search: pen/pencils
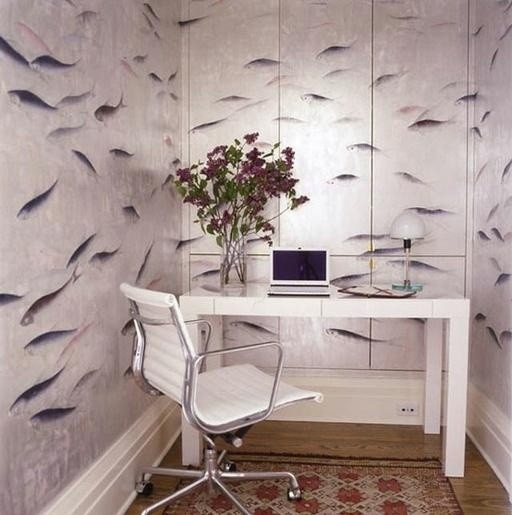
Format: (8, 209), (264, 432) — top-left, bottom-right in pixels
(373, 286), (392, 294)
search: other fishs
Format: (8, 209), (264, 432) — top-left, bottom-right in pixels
(0, 0), (512, 432)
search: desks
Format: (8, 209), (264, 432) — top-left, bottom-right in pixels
(178, 280), (472, 479)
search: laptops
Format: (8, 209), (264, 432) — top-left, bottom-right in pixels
(267, 247), (330, 296)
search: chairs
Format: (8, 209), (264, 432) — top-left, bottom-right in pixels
(117, 284), (326, 515)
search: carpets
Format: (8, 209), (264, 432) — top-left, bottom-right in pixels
(163, 448), (464, 515)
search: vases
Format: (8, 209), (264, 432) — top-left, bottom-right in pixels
(220, 239), (248, 285)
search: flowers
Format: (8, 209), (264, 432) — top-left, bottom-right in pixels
(172, 131), (310, 282)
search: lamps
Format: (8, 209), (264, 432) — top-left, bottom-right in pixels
(388, 212), (428, 291)
